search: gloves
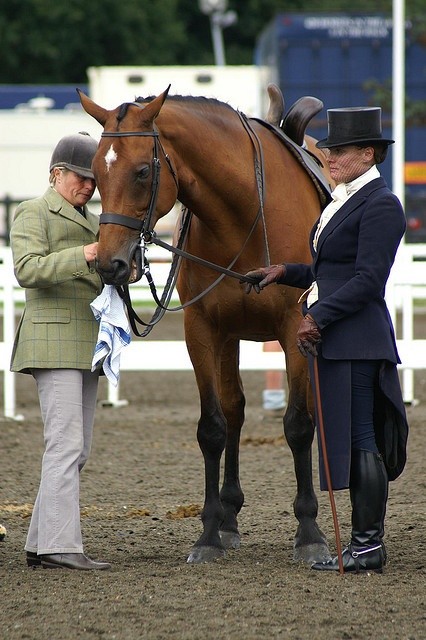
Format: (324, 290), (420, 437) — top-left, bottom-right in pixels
(295, 314), (321, 360)
(239, 264), (287, 295)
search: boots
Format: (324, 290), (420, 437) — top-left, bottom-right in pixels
(381, 462), (388, 566)
(312, 449), (386, 575)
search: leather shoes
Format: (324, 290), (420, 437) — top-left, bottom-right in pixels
(26, 551), (42, 567)
(40, 554), (112, 572)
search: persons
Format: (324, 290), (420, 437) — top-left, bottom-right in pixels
(240, 107), (410, 574)
(9, 130), (111, 572)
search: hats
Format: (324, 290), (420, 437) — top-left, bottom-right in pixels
(315, 106), (394, 150)
(48, 131), (99, 178)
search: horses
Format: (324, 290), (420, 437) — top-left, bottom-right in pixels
(75, 84), (339, 564)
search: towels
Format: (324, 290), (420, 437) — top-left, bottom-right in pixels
(90, 285), (133, 387)
